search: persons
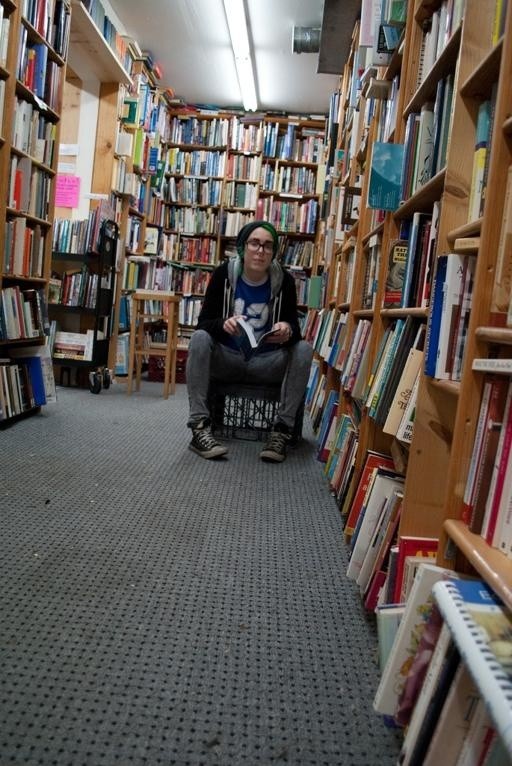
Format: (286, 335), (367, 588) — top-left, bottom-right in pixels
(185, 221), (313, 463)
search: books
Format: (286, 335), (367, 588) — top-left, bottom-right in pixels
(1, 0), (177, 419)
(178, 99), (307, 357)
(306, 0), (512, 766)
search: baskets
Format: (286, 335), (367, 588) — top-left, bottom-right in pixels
(208, 382), (308, 448)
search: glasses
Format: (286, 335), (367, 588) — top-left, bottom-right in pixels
(246, 240), (274, 253)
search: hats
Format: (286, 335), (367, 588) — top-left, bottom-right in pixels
(236, 221), (279, 260)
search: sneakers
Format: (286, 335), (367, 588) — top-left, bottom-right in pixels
(260, 423), (293, 462)
(189, 419), (229, 459)
